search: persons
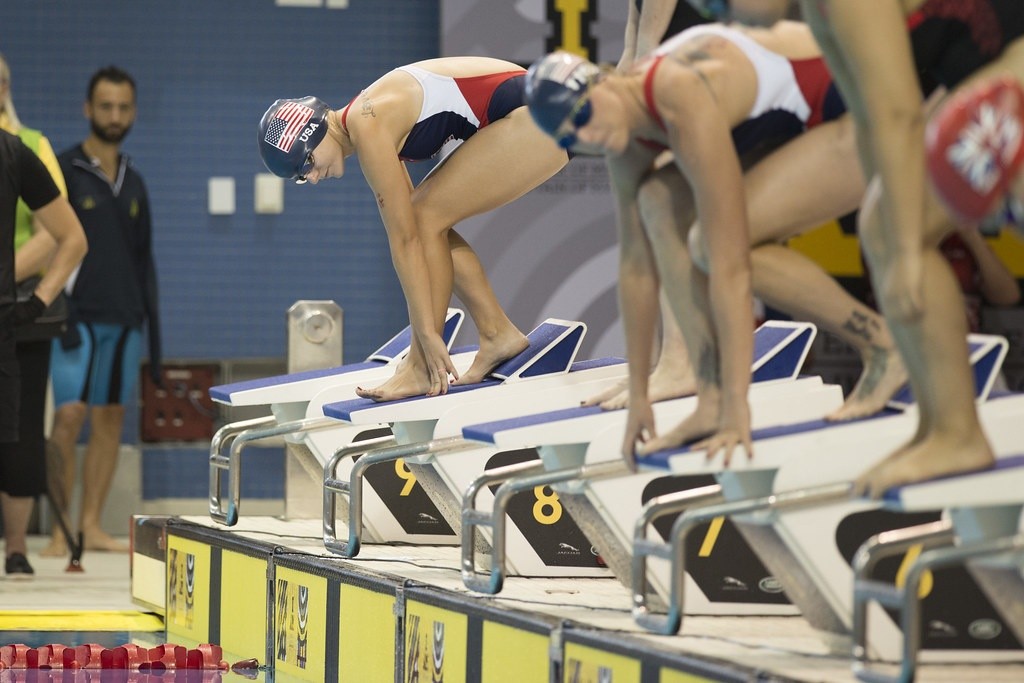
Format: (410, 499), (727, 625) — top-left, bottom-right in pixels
(578, 0), (803, 409)
(257, 55), (572, 403)
(41, 64), (160, 557)
(687, 0), (1024, 500)
(0, 56), (88, 579)
(525, 19), (909, 466)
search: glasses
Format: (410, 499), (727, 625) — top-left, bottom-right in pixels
(296, 154), (315, 186)
(559, 99), (592, 148)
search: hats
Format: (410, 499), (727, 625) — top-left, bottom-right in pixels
(256, 96), (331, 179)
(522, 48), (599, 139)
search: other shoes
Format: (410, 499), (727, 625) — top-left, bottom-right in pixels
(4, 552), (33, 582)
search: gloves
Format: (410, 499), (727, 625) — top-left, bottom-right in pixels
(0, 292), (47, 328)
